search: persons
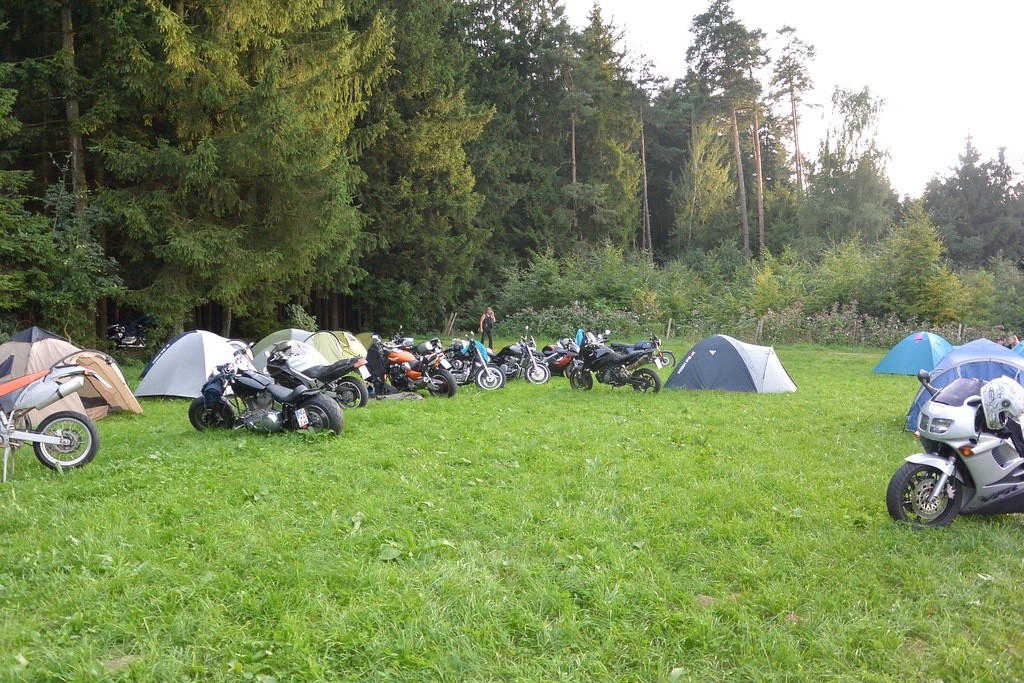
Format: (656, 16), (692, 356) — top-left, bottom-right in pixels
(480, 307), (497, 349)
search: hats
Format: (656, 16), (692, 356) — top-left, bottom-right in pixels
(995, 336), (1003, 340)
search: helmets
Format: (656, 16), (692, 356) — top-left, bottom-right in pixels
(980, 375), (1024, 430)
(455, 342), (462, 351)
(431, 338), (443, 348)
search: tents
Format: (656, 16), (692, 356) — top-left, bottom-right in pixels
(0, 326), (144, 423)
(903, 338), (1024, 433)
(871, 331), (955, 377)
(251, 328), (382, 373)
(132, 329), (258, 399)
(663, 334), (799, 395)
(1011, 339), (1024, 359)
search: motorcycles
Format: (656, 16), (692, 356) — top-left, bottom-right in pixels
(414, 331), (506, 390)
(264, 341), (368, 410)
(0, 364), (112, 483)
(486, 326), (551, 385)
(886, 369), (1024, 530)
(544, 327), (611, 379)
(389, 325), (442, 359)
(372, 335), (457, 398)
(569, 329), (676, 396)
(188, 342), (344, 438)
(611, 332), (676, 369)
